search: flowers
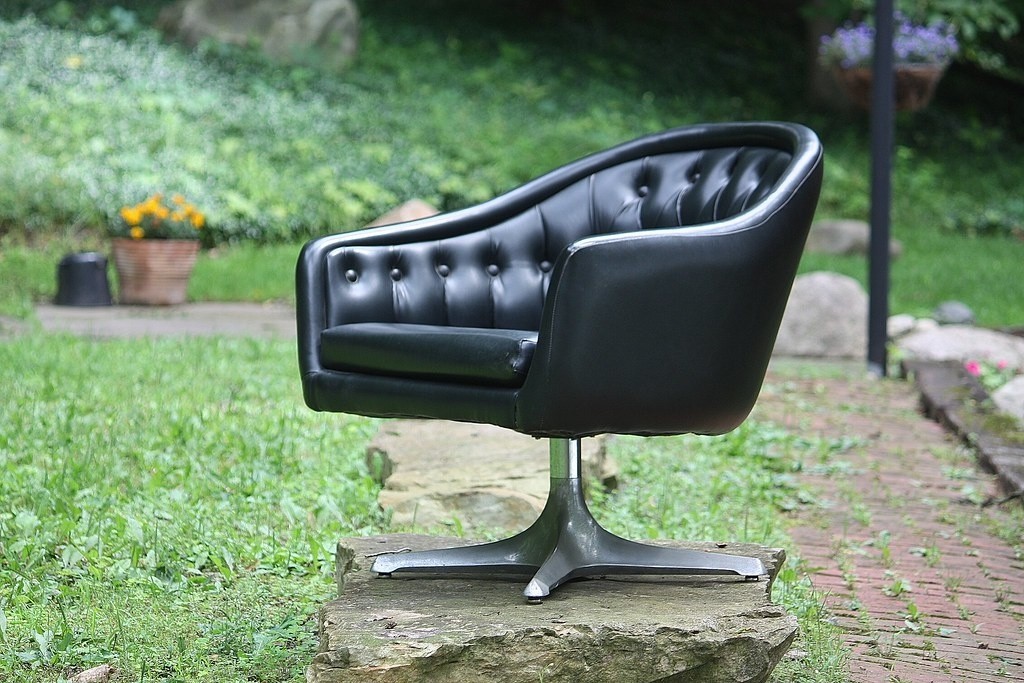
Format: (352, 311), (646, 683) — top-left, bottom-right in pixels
(820, 22), (958, 68)
(102, 184), (209, 240)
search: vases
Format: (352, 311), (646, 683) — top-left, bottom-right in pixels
(111, 237), (202, 303)
(831, 67), (936, 111)
(54, 255), (113, 305)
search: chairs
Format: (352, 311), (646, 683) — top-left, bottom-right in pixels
(295, 121), (823, 603)
